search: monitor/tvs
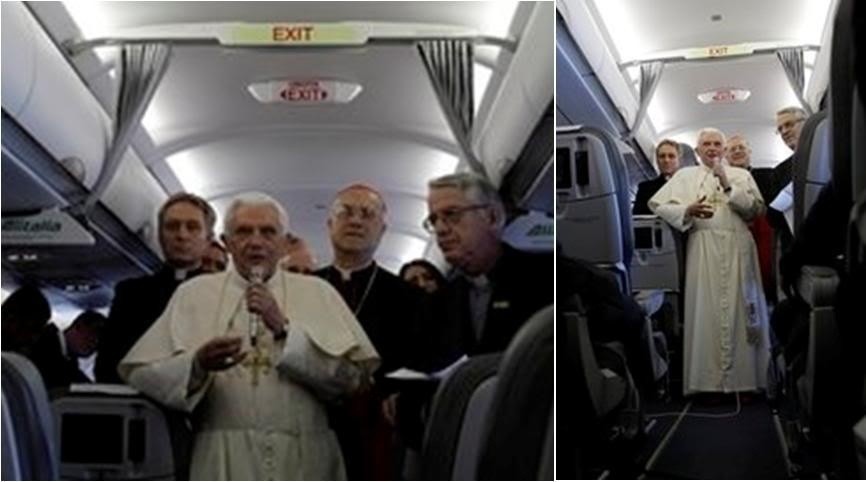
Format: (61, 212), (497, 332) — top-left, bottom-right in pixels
(57, 410), (127, 469)
(555, 143), (573, 190)
(633, 225), (653, 250)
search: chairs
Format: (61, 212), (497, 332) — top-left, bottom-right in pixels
(2, 350), (61, 481)
(421, 306), (555, 480)
(47, 380), (191, 481)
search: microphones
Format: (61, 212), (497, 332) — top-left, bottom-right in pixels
(245, 268), (265, 343)
(712, 157), (721, 190)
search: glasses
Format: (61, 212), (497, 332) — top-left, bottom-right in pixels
(423, 204), (489, 234)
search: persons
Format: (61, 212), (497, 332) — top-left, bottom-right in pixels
(633, 138), (683, 251)
(770, 107), (808, 180)
(1, 173), (552, 481)
(727, 135), (790, 256)
(648, 128), (772, 414)
